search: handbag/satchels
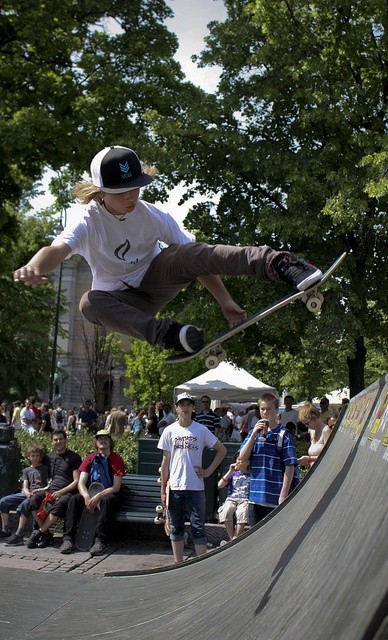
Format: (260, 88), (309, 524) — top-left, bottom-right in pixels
(36, 491), (56, 522)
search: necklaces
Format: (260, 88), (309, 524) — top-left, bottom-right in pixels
(104, 203), (126, 221)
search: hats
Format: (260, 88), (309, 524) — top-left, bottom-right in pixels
(90, 146), (152, 195)
(175, 391), (195, 405)
(96, 430), (111, 437)
(233, 449), (252, 458)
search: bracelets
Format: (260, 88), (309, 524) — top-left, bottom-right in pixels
(307, 456), (311, 464)
(221, 477), (228, 482)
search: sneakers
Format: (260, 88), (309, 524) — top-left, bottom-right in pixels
(163, 322), (204, 353)
(36, 533), (54, 548)
(26, 530), (41, 547)
(0, 530), (11, 538)
(2, 533), (25, 545)
(277, 254), (323, 291)
(59, 534), (75, 554)
(89, 538), (110, 556)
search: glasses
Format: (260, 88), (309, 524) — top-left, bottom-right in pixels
(202, 400), (209, 404)
(305, 420), (311, 425)
(85, 403), (90, 406)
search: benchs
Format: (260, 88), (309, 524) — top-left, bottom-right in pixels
(114, 474), (160, 525)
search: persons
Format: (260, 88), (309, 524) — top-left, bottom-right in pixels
(318, 397), (333, 424)
(14, 146), (323, 354)
(0, 445), (50, 546)
(285, 422), (298, 440)
(297, 422), (310, 443)
(327, 414), (337, 431)
(194, 395), (221, 437)
(59, 429), (126, 556)
(278, 396), (299, 435)
(24, 429), (82, 548)
(214, 403), (259, 439)
(218, 449), (252, 542)
(237, 393), (299, 528)
(157, 392), (227, 564)
(297, 401), (331, 468)
(0, 395), (179, 437)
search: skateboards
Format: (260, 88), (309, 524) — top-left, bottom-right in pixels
(153, 466), (171, 537)
(75, 481), (105, 552)
(159, 251), (348, 366)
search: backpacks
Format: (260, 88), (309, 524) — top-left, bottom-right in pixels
(90, 453), (112, 489)
(56, 410), (62, 423)
(277, 428), (299, 494)
(224, 416), (233, 437)
